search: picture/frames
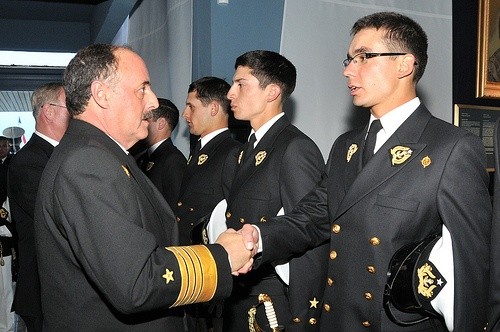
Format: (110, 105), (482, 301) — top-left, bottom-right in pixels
(454, 104), (500, 173)
(476, 0), (500, 100)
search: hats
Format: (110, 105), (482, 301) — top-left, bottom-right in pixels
(382, 224), (457, 332)
(271, 206), (294, 285)
(3, 127), (25, 138)
(189, 199), (229, 246)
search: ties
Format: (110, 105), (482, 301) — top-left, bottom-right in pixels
(243, 133), (257, 163)
(193, 141), (201, 158)
(362, 120), (383, 169)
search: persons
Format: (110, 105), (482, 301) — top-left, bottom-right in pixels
(174, 76), (245, 332)
(2, 127), (25, 154)
(232, 12), (493, 332)
(213, 50), (326, 332)
(0, 136), (11, 205)
(483, 117), (500, 332)
(34, 43), (258, 332)
(128, 97), (188, 215)
(7, 83), (81, 332)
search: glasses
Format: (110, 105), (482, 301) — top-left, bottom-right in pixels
(342, 53), (418, 70)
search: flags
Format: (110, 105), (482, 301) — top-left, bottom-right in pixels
(17, 117), (26, 143)
